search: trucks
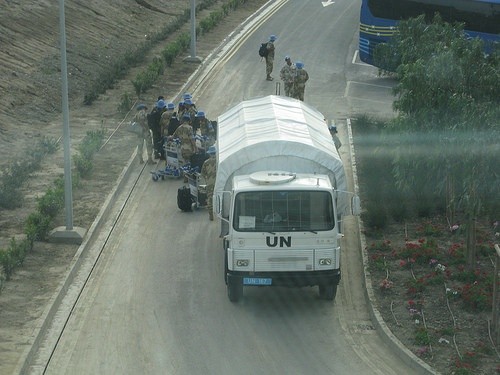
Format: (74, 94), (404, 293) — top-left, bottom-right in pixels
(212, 95), (362, 302)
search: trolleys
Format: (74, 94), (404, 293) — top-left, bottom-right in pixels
(185, 169), (207, 208)
(151, 142), (181, 181)
(183, 137), (206, 184)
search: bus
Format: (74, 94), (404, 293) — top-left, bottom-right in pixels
(359, 0), (500, 76)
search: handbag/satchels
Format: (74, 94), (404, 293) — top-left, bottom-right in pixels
(127, 121), (143, 136)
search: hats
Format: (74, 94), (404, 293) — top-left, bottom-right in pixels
(329, 126), (337, 132)
(206, 146), (216, 154)
(179, 101), (186, 106)
(182, 93), (193, 98)
(137, 103), (148, 111)
(185, 99), (192, 105)
(269, 35), (277, 41)
(157, 100), (166, 108)
(285, 56), (290, 62)
(167, 103), (176, 109)
(296, 62), (304, 67)
(195, 111), (206, 117)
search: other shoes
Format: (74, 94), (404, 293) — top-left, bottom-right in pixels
(210, 218), (213, 221)
(266, 76), (274, 81)
(140, 161), (144, 164)
(155, 154), (160, 159)
(184, 177), (188, 183)
(148, 160), (157, 165)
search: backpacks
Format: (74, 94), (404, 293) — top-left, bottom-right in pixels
(259, 43), (268, 57)
(161, 111), (171, 129)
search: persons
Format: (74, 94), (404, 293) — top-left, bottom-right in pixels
(169, 116), (199, 183)
(148, 93), (202, 160)
(279, 55), (298, 98)
(264, 35), (278, 81)
(291, 62), (310, 102)
(201, 146), (218, 222)
(130, 103), (157, 165)
(194, 111), (215, 158)
(328, 124), (342, 150)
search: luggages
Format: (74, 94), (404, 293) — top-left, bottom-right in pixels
(177, 185), (191, 210)
